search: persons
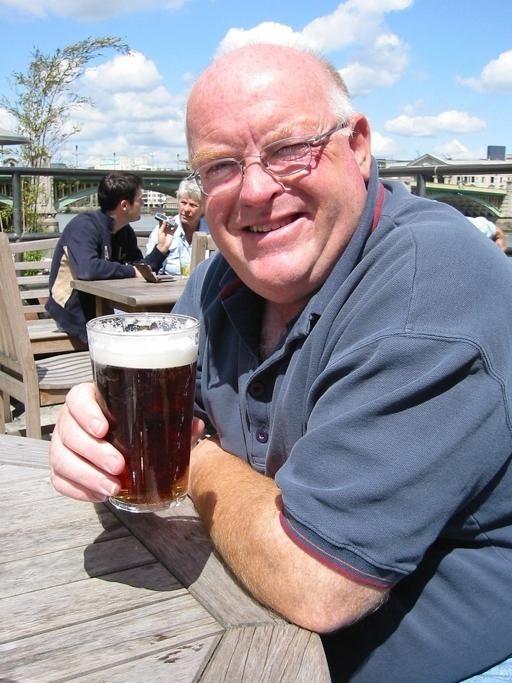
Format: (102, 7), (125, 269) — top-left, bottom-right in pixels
(463, 214), (508, 252)
(46, 39), (511, 683)
(40, 168), (178, 353)
(144, 174), (207, 277)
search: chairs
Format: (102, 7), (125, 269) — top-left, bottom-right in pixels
(190, 231), (218, 274)
(0, 233), (94, 440)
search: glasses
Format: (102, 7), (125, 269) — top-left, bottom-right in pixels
(186, 119), (352, 197)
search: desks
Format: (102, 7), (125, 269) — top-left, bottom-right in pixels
(0, 434), (332, 683)
(69, 274), (191, 318)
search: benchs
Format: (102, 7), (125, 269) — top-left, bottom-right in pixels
(9, 237), (150, 360)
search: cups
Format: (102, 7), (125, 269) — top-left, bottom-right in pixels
(86, 313), (201, 513)
(179, 246), (192, 275)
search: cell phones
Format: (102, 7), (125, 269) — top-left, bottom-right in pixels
(154, 212), (176, 227)
